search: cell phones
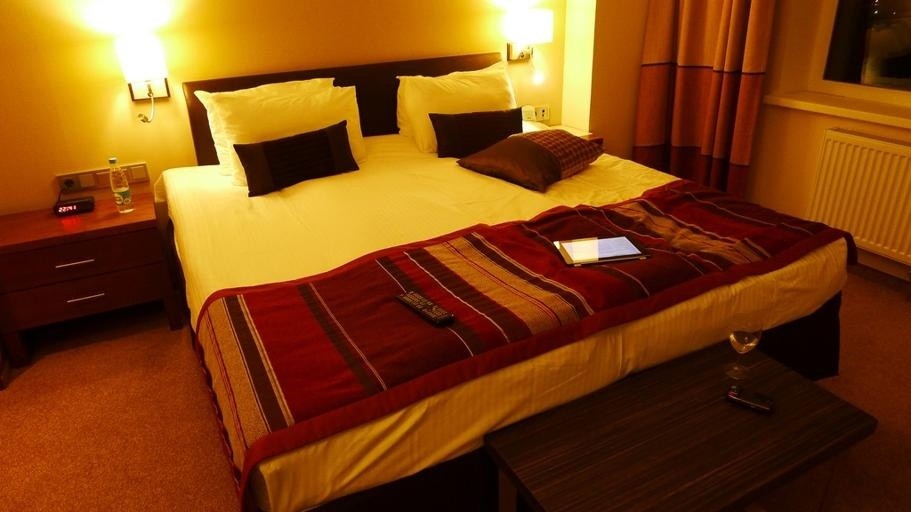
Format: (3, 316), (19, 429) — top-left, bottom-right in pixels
(726, 385), (776, 412)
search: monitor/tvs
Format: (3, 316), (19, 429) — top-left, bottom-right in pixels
(823, 0), (911, 93)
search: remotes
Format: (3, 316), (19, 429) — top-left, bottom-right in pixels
(396, 291), (455, 327)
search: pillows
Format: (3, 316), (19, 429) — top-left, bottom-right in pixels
(211, 86), (366, 185)
(429, 108), (523, 159)
(394, 59), (516, 155)
(458, 127), (607, 192)
(194, 78), (335, 180)
(232, 119), (360, 198)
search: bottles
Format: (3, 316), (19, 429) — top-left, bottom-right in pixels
(105, 157), (135, 213)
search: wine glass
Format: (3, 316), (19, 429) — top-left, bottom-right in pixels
(723, 310), (765, 382)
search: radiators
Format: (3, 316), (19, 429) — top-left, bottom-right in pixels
(809, 128), (911, 267)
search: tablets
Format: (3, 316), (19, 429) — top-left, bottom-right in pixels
(553, 235), (650, 267)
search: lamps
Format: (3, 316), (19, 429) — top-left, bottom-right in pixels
(116, 40), (171, 127)
(502, 11), (557, 63)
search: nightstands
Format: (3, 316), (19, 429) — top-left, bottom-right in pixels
(0, 194), (183, 367)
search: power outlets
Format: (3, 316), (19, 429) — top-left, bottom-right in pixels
(58, 173), (80, 196)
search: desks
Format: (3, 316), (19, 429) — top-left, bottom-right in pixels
(486, 345), (880, 512)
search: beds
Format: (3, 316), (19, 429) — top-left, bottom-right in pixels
(154, 51), (860, 512)
